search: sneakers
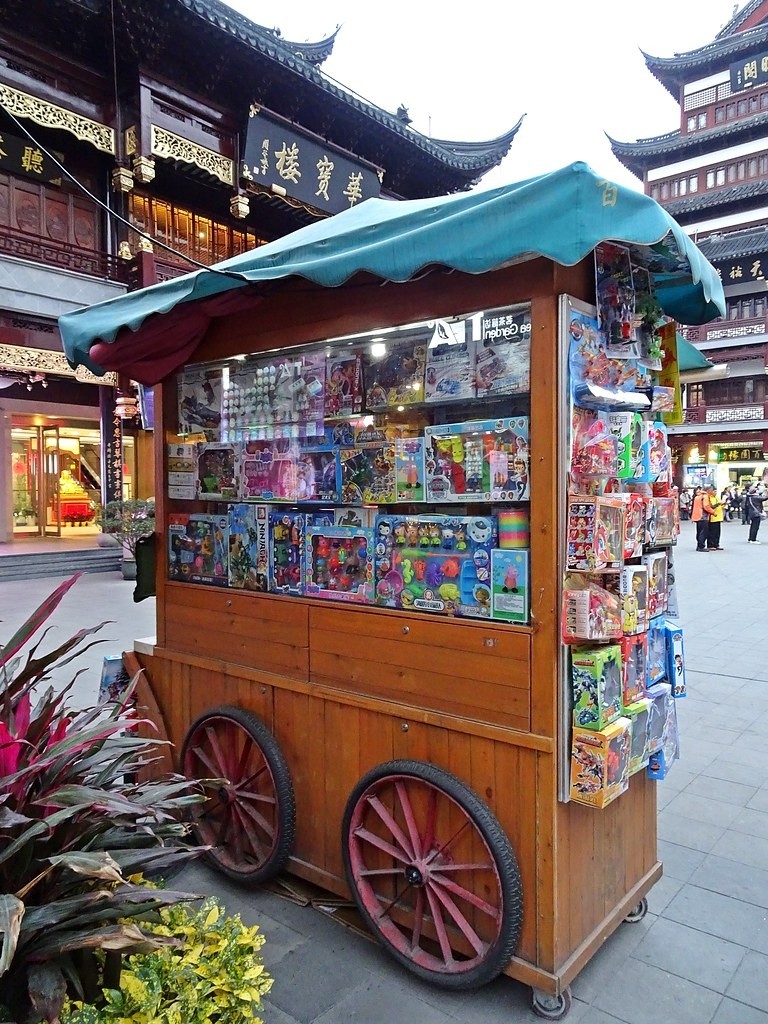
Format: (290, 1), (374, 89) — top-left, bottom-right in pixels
(696, 547), (710, 552)
(750, 540), (761, 545)
(707, 547), (716, 551)
(747, 539), (759, 543)
(716, 547), (724, 550)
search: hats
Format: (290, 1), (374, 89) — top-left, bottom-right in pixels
(745, 484), (752, 490)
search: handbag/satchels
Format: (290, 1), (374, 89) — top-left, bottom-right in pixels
(759, 510), (768, 520)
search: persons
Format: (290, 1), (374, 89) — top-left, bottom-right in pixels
(691, 486), (717, 552)
(721, 483), (768, 545)
(705, 484), (729, 551)
(679, 486), (702, 520)
